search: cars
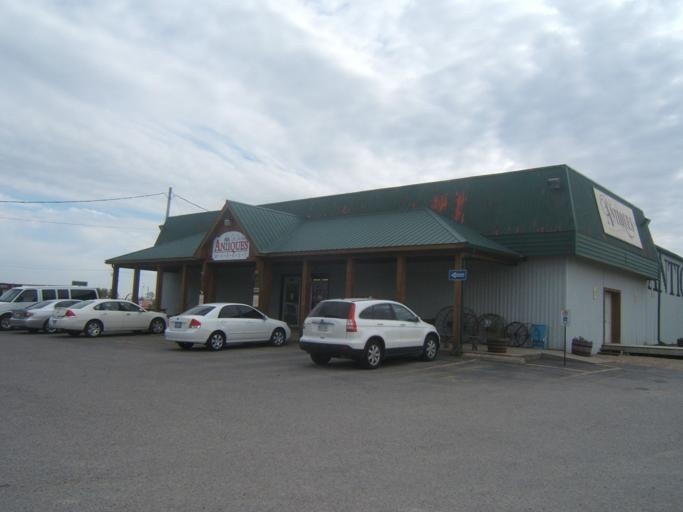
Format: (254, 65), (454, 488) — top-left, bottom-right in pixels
(49, 298), (168, 341)
(10, 299), (83, 332)
(162, 299), (290, 355)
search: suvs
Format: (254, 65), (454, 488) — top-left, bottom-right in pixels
(296, 295), (446, 366)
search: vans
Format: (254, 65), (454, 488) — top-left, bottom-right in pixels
(0, 285), (98, 331)
